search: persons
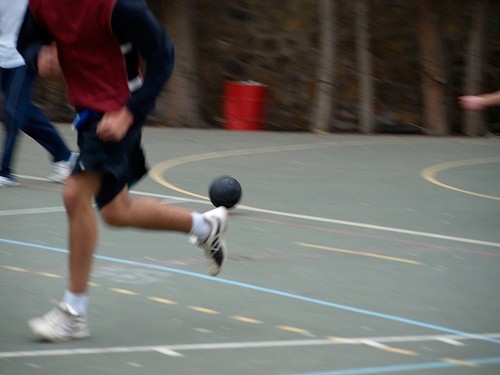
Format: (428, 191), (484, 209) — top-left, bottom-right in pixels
(458, 91), (500, 112)
(0, 0), (78, 188)
(16, 0), (228, 342)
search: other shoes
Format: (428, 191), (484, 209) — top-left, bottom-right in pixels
(0, 175), (21, 188)
(49, 152), (80, 183)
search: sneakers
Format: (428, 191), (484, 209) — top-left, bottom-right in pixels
(25, 297), (91, 340)
(191, 205), (228, 275)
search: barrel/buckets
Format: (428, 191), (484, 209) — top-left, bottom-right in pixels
(223, 79), (266, 128)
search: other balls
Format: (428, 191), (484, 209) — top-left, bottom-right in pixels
(209, 175), (241, 209)
